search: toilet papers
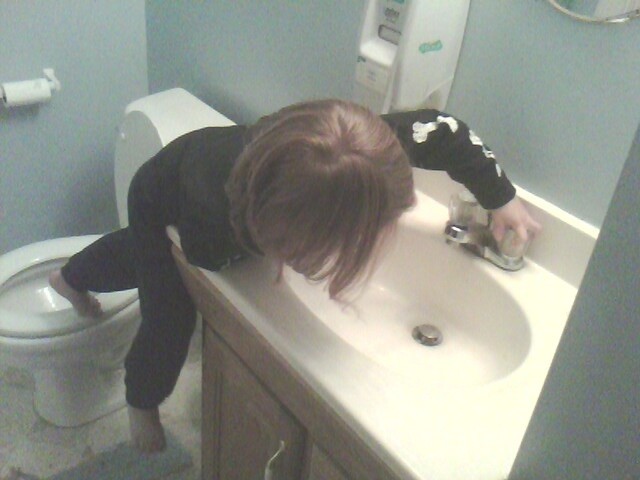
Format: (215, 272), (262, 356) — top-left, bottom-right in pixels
(0, 68), (61, 107)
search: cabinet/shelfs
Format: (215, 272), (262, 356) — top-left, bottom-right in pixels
(202, 322), (400, 480)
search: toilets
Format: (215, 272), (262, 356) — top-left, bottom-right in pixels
(0, 86), (237, 428)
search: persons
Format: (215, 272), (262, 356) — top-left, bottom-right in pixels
(49, 97), (543, 459)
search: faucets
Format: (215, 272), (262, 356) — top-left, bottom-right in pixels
(444, 198), (529, 270)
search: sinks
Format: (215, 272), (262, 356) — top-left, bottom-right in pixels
(278, 216), (534, 385)
